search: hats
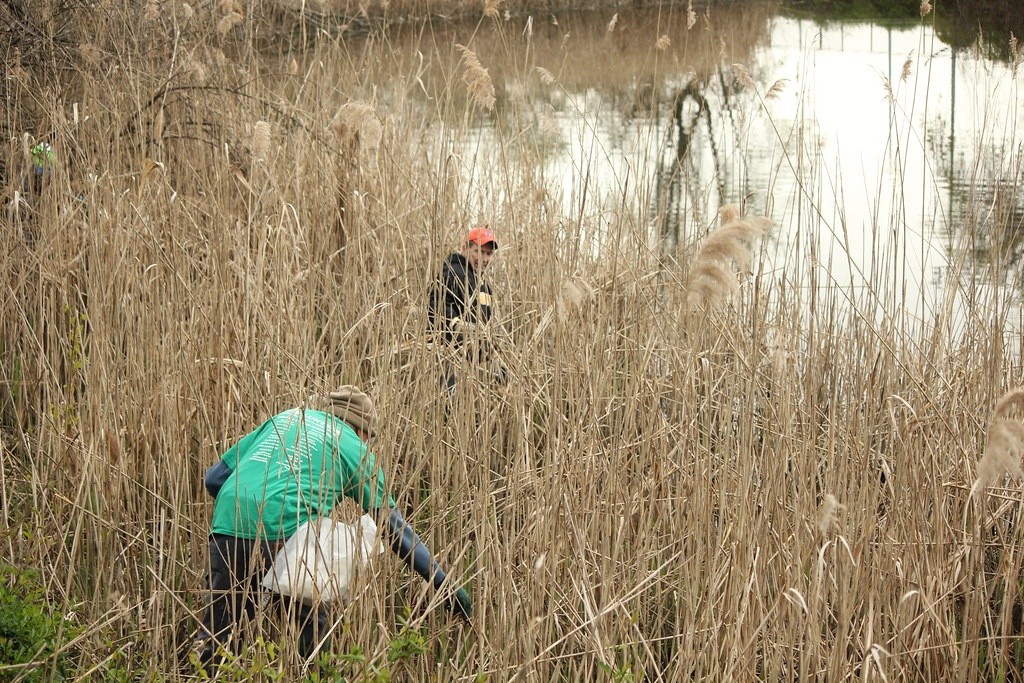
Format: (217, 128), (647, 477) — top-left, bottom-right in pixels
(329, 384), (378, 436)
(465, 228), (498, 250)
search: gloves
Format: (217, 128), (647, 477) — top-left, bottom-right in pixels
(438, 578), (474, 620)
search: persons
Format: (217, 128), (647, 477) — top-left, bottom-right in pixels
(194, 386), (471, 683)
(27, 142), (63, 203)
(392, 229), (508, 519)
